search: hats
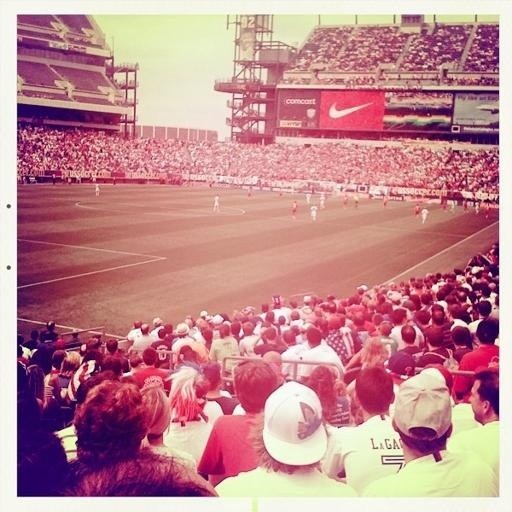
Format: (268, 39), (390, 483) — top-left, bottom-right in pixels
(392, 367), (452, 441)
(262, 381), (328, 466)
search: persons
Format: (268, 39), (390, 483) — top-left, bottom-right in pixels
(427, 242), (499, 292)
(17, 122), (295, 187)
(290, 141), (499, 224)
(260, 277), (499, 365)
(17, 309), (499, 495)
(213, 194), (221, 212)
(284, 23), (498, 86)
(95, 183), (101, 196)
